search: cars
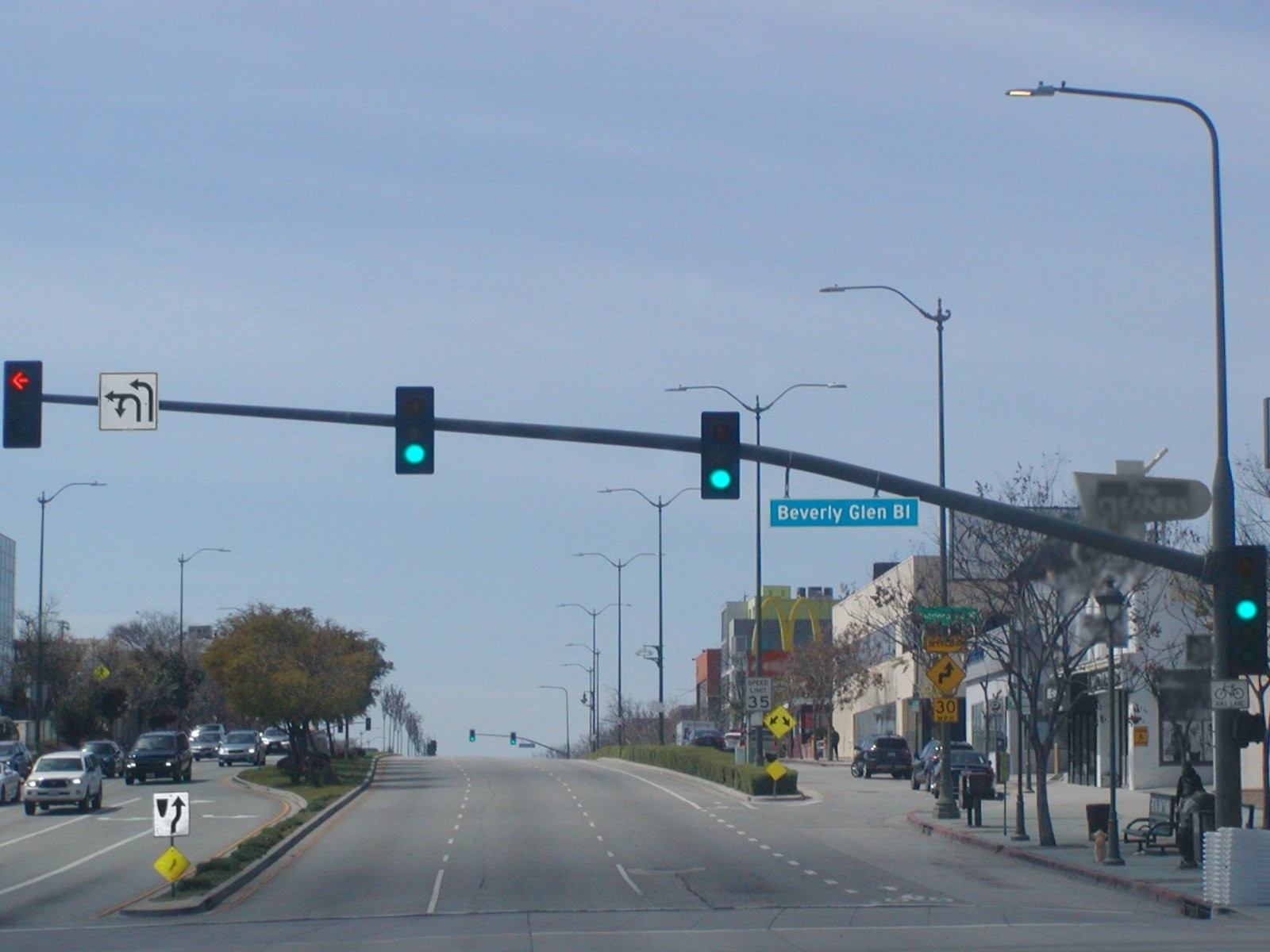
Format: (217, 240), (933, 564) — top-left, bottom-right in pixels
(686, 729), (727, 752)
(0, 723), (292, 817)
(848, 732), (997, 805)
(723, 733), (740, 750)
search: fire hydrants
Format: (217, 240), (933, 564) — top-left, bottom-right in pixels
(1091, 829), (1110, 862)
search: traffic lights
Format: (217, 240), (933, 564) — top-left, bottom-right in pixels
(510, 732), (516, 745)
(700, 411), (743, 502)
(470, 729), (475, 742)
(1, 360), (44, 449)
(1212, 545), (1270, 679)
(395, 386), (436, 475)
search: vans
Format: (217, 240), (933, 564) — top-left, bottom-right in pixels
(747, 728), (777, 758)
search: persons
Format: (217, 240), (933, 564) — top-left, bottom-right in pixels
(1174, 762), (1206, 820)
(1171, 723), (1203, 762)
(829, 727), (840, 760)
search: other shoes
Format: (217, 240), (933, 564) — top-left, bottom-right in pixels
(1178, 860), (1198, 869)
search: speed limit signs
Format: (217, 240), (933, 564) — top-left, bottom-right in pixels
(745, 678), (771, 711)
(933, 697), (961, 724)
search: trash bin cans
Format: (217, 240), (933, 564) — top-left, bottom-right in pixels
(958, 770), (974, 808)
(1086, 804), (1110, 841)
(1192, 811), (1215, 863)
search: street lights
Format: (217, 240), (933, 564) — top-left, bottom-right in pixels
(573, 552), (667, 748)
(177, 547), (232, 734)
(666, 383), (849, 772)
(820, 283), (962, 822)
(1089, 572), (1132, 865)
(538, 603), (633, 752)
(597, 488), (701, 747)
(1005, 80), (1241, 838)
(1009, 610), (1033, 840)
(35, 481), (108, 750)
